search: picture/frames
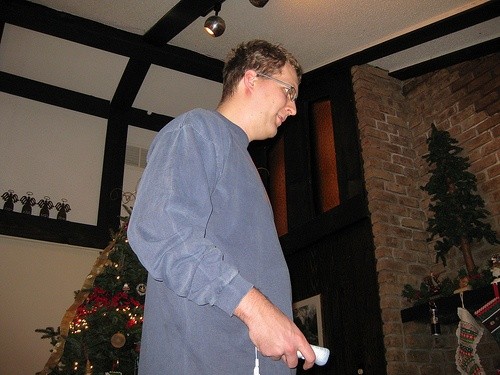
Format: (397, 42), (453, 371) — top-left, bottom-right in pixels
(293, 294), (324, 348)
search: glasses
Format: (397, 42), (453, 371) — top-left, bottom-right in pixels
(256, 72), (297, 102)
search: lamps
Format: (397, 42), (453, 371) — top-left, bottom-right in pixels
(204, 2), (226, 38)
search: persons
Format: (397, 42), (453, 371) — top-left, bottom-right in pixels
(127, 40), (315, 375)
